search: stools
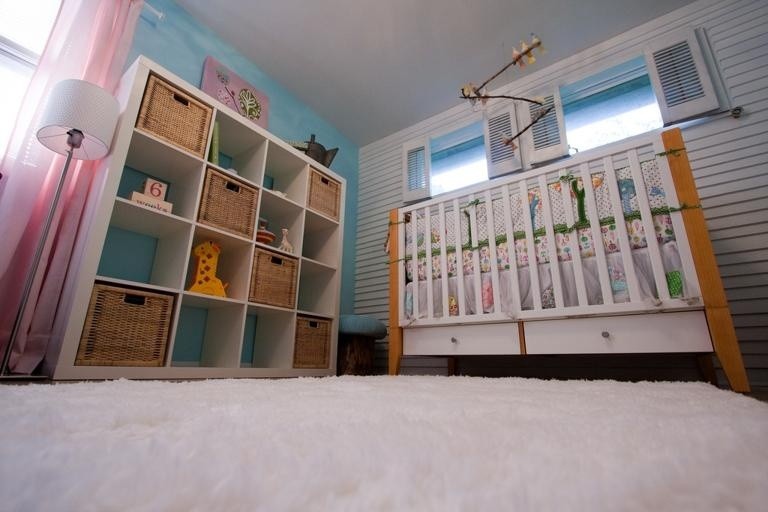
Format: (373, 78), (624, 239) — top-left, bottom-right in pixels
(338, 313), (387, 374)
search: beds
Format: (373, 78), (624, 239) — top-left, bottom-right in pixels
(384, 127), (751, 392)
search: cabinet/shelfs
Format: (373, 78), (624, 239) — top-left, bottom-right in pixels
(44, 55), (349, 378)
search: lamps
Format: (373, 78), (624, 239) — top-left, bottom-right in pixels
(3, 77), (124, 379)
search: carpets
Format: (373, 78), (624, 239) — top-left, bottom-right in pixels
(2, 371), (768, 512)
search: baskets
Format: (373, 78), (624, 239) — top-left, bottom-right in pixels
(306, 165), (340, 223)
(197, 168), (259, 241)
(293, 316), (332, 369)
(249, 247), (299, 310)
(135, 75), (214, 159)
(75, 283), (174, 368)
